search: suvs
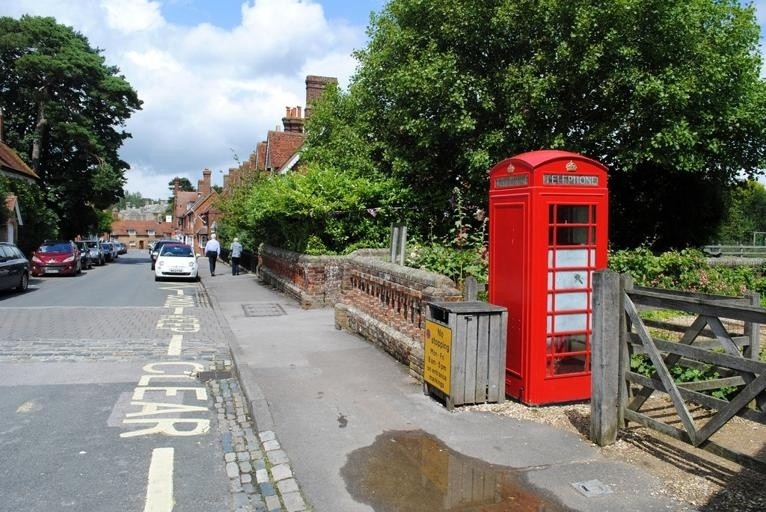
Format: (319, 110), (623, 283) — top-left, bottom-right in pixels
(0, 241), (31, 295)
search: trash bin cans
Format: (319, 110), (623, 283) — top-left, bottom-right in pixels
(423, 301), (508, 410)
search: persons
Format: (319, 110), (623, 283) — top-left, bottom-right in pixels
(204, 233), (220, 276)
(229, 238), (242, 275)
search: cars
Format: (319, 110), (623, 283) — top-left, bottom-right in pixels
(148, 238), (199, 282)
(30, 237), (128, 278)
